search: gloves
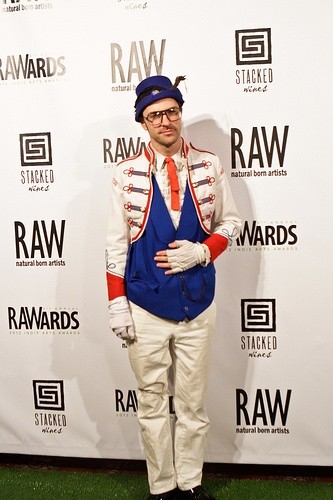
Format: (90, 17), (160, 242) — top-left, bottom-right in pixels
(109, 301), (134, 340)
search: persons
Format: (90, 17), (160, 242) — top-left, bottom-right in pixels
(105, 76), (241, 500)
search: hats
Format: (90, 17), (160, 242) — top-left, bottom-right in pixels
(134, 76), (184, 122)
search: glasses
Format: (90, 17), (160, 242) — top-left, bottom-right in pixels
(143, 107), (182, 124)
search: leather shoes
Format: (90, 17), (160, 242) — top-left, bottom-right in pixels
(177, 486), (216, 500)
(148, 487), (180, 500)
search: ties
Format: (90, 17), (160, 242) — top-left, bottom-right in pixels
(164, 158), (180, 211)
